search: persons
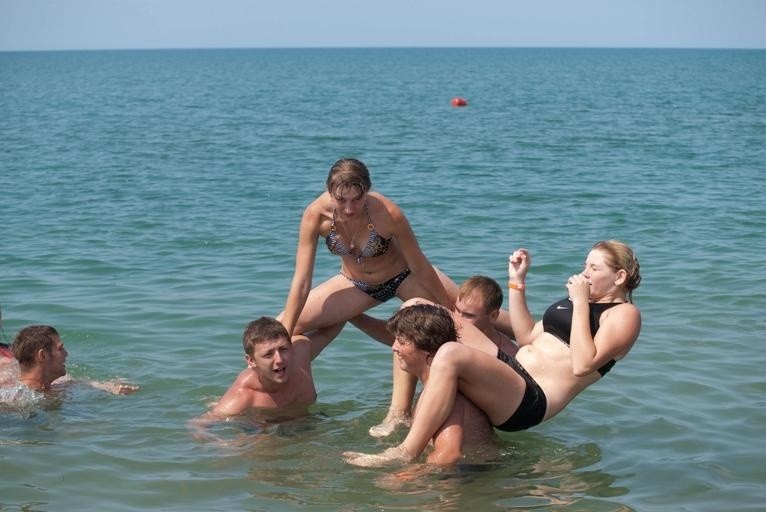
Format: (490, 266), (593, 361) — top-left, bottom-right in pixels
(188, 316), (348, 458)
(0, 322), (141, 419)
(342, 235), (643, 470)
(375, 299), (507, 490)
(346, 277), (519, 363)
(244, 156), (518, 365)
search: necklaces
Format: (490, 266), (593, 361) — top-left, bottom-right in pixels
(339, 216), (364, 251)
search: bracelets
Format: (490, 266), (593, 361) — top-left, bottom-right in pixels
(504, 281), (526, 292)
